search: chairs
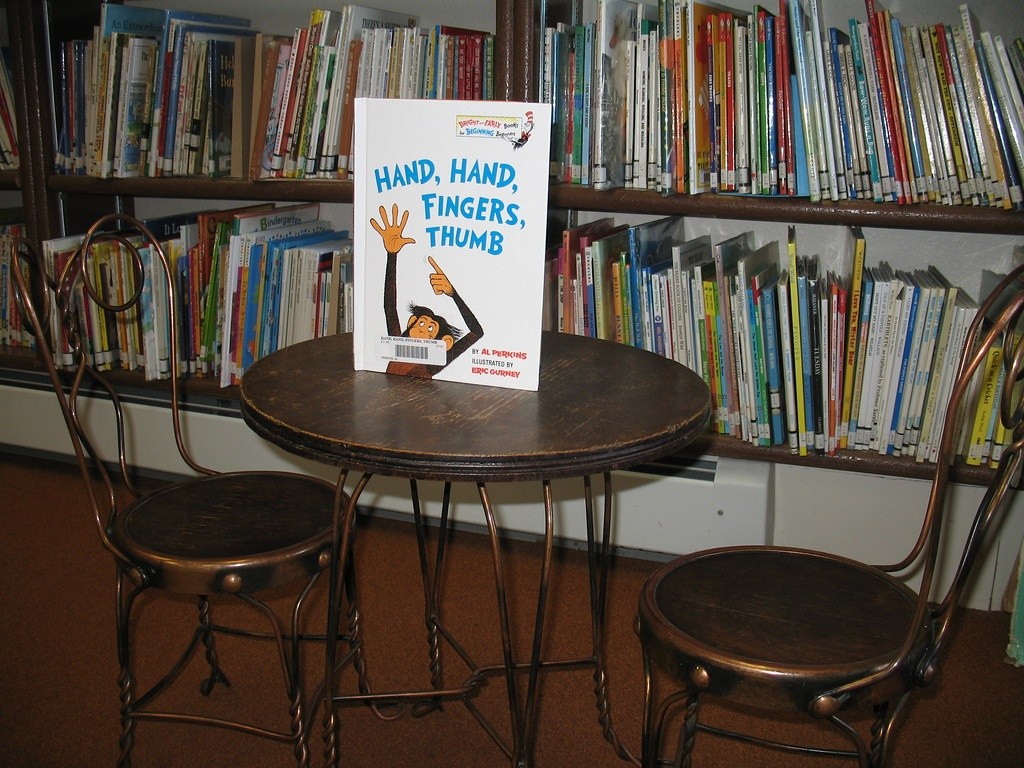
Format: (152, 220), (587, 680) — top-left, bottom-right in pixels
(632, 264), (1024, 768)
(11, 212), (406, 768)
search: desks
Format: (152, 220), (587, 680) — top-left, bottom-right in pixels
(238, 330), (712, 768)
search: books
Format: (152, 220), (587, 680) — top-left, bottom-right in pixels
(353, 98), (551, 392)
(0, 201), (355, 388)
(543, 216), (1024, 470)
(0, 0), (496, 182)
(538, 0), (1024, 211)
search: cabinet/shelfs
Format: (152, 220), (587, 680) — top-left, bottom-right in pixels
(0, 0), (1024, 492)
